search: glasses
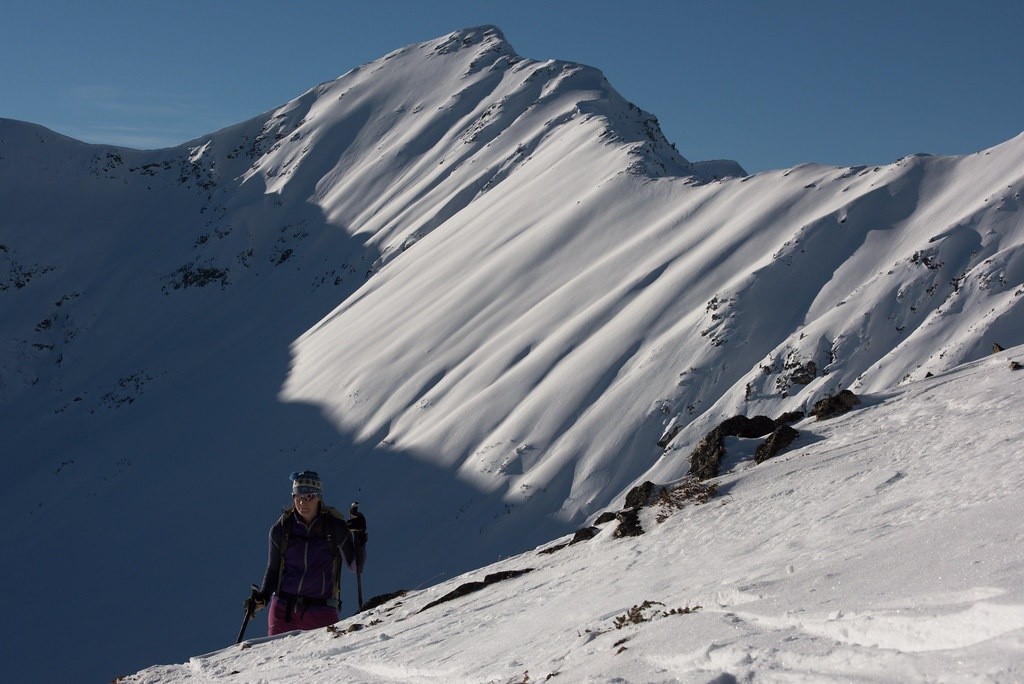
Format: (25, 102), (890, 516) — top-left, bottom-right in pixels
(293, 493), (317, 501)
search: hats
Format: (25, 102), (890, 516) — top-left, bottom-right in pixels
(289, 470), (323, 497)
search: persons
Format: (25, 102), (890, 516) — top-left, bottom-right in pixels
(243, 470), (369, 640)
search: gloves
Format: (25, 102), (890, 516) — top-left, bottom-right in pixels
(243, 595), (266, 619)
(345, 508), (369, 545)
